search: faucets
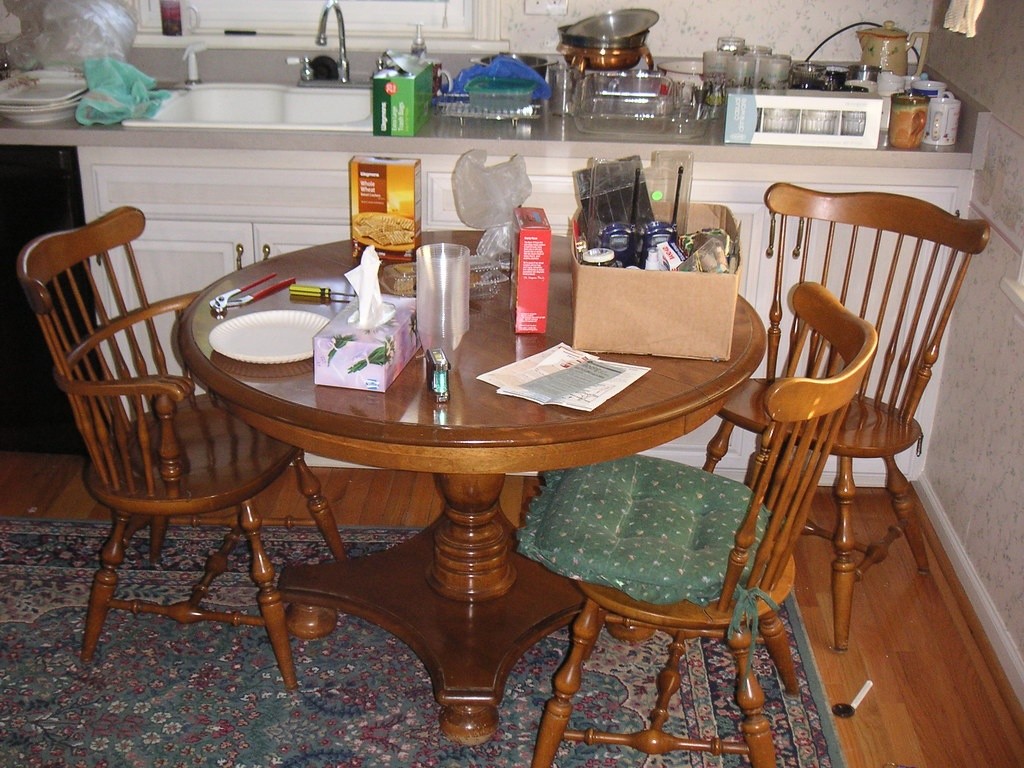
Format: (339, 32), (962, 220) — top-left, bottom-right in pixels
(286, 0), (387, 90)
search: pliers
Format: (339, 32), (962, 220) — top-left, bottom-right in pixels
(208, 272), (295, 313)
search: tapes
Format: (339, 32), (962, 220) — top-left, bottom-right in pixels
(583, 247), (615, 263)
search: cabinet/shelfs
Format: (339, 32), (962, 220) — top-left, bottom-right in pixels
(76, 145), (976, 488)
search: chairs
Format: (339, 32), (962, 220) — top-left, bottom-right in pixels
(701, 182), (990, 651)
(17, 206), (346, 694)
(517, 279), (878, 768)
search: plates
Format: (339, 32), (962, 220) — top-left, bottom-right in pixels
(351, 212), (414, 251)
(0, 70), (88, 124)
(209, 310), (331, 365)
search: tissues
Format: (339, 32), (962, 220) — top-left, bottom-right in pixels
(312, 241), (426, 394)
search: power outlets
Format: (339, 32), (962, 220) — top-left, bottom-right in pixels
(524, 0), (567, 16)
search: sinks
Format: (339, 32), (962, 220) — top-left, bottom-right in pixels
(121, 82), (374, 132)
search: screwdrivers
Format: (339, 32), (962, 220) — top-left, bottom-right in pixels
(290, 285), (356, 300)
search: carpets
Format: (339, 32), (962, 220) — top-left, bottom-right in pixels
(0, 512), (845, 767)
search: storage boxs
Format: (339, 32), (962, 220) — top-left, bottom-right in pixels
(371, 47), (435, 136)
(508, 206), (553, 335)
(568, 200), (742, 360)
(345, 156), (422, 266)
(721, 87), (883, 152)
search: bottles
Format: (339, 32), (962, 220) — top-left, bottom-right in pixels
(0, 43), (21, 81)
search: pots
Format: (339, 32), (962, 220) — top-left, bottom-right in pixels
(556, 42), (646, 71)
(557, 25), (650, 48)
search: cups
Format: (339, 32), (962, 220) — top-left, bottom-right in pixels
(160, 0), (199, 36)
(793, 62), (880, 91)
(416, 242), (470, 372)
(877, 72), (961, 149)
(703, 38), (791, 119)
(423, 58), (453, 98)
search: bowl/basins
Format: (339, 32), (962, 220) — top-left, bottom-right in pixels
(470, 54), (558, 81)
(464, 77), (537, 110)
(657, 61), (703, 86)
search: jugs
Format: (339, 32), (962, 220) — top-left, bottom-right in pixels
(856, 21), (933, 77)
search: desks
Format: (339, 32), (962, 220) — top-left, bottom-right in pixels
(171, 231), (765, 747)
